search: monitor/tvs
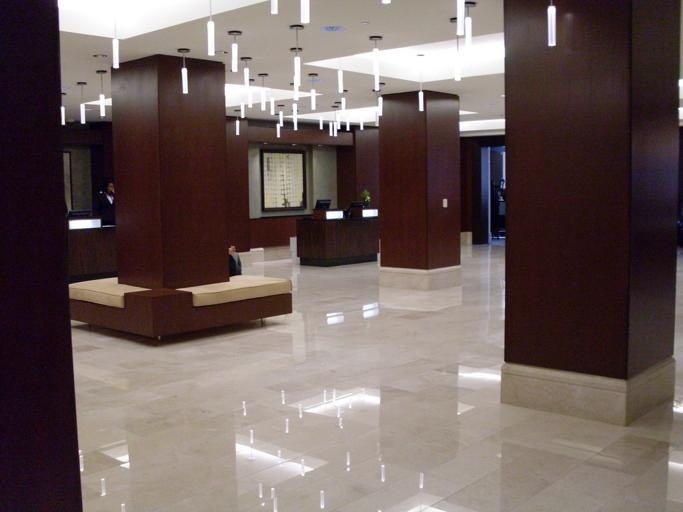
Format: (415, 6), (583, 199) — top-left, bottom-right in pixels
(315, 200), (331, 210)
(69, 210), (91, 219)
(352, 202), (362, 208)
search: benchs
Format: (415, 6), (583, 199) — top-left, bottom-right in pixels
(67, 276), (293, 344)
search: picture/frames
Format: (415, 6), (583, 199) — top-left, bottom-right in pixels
(259, 148), (306, 211)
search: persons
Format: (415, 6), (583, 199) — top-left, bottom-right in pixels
(92, 180), (115, 226)
